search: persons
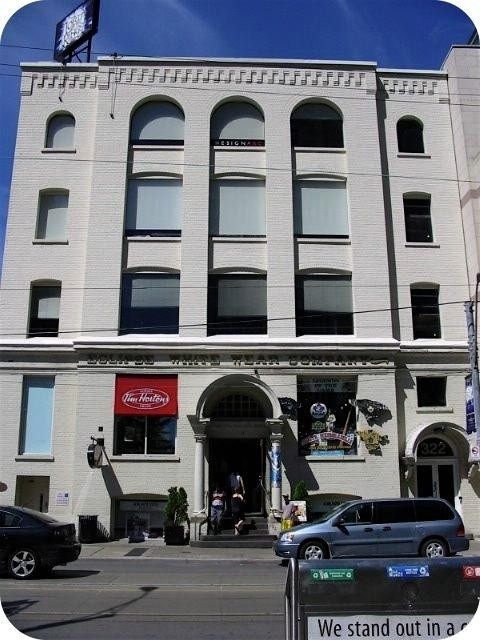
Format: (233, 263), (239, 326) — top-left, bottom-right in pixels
(211, 470), (246, 537)
(269, 493), (303, 531)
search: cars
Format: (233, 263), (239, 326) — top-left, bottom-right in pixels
(0, 506), (81, 578)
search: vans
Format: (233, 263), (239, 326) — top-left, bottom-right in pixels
(272, 498), (469, 560)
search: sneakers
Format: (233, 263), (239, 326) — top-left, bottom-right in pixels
(234, 525), (241, 536)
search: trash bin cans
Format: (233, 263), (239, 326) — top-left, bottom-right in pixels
(283, 558), (480, 640)
(78, 515), (99, 544)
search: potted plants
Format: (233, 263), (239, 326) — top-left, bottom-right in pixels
(163, 485), (190, 545)
